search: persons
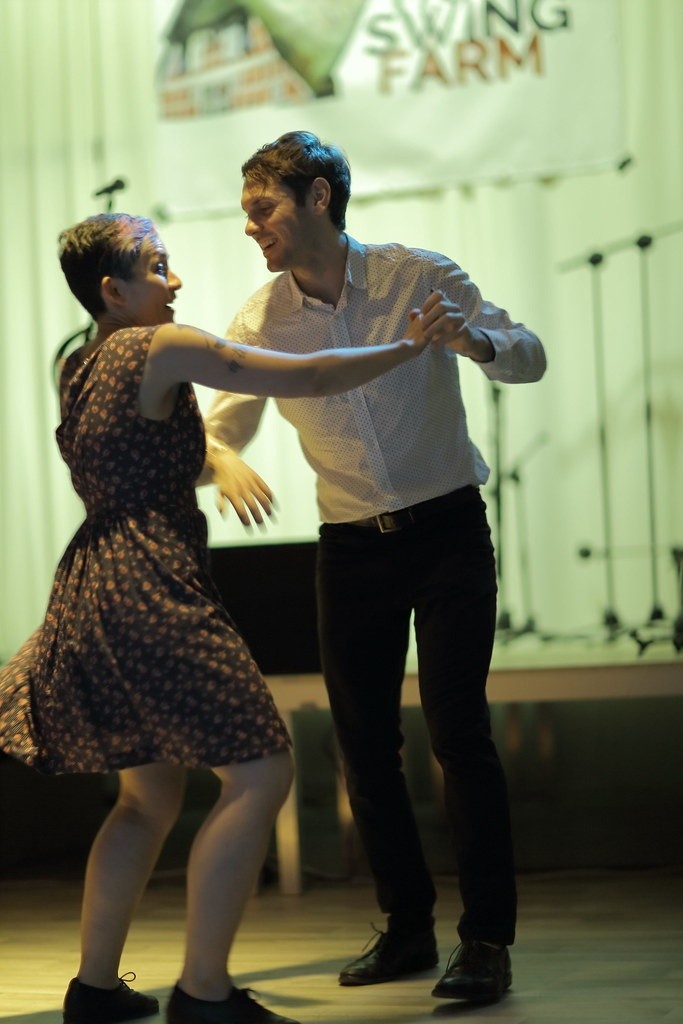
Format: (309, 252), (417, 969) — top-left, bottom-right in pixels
(0, 213), (454, 1024)
(206, 131), (547, 1002)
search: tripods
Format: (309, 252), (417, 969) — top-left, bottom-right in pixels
(482, 224), (683, 658)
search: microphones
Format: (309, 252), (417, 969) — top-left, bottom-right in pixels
(97, 180), (125, 198)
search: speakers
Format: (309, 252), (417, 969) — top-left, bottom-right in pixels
(204, 541), (325, 676)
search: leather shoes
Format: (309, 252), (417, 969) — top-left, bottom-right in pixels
(432, 938), (515, 1001)
(339, 909), (439, 985)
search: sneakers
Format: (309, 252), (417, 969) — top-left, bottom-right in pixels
(64, 974), (157, 1023)
(167, 981), (298, 1024)
